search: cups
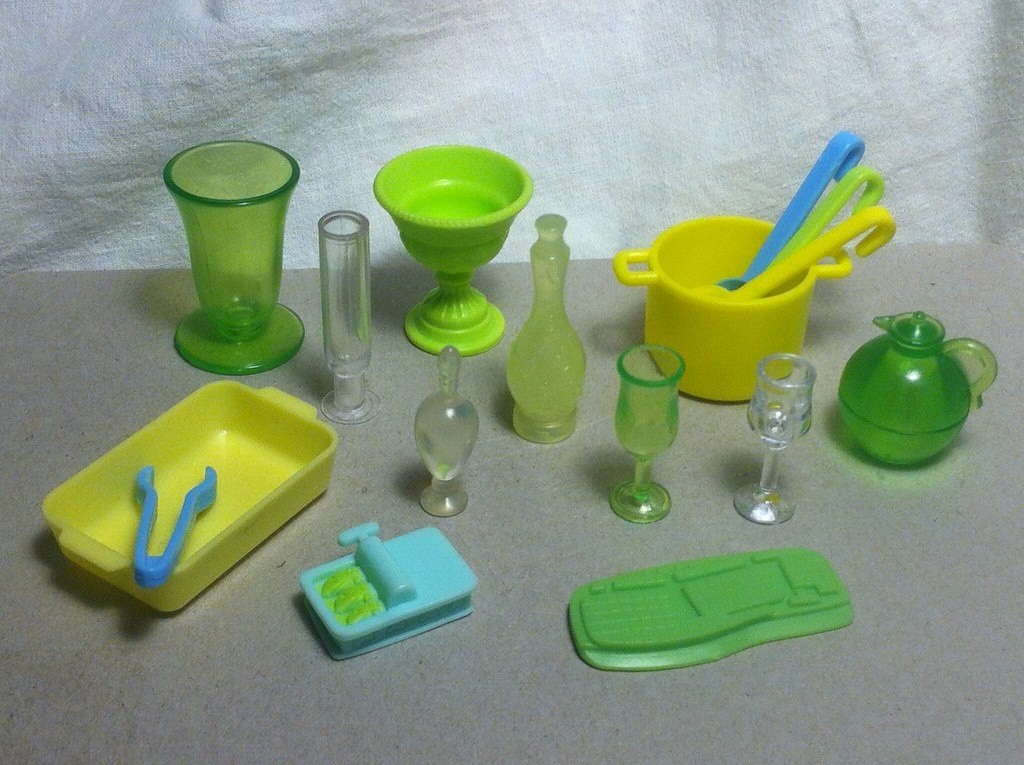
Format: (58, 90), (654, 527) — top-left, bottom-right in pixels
(160, 139), (307, 375)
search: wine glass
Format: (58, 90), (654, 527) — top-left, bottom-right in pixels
(371, 143), (534, 356)
(607, 342), (686, 526)
(317, 208), (384, 425)
(733, 349), (820, 526)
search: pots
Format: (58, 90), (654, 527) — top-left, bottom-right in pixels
(608, 213), (853, 403)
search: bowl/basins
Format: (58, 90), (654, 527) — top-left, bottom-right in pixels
(38, 377), (339, 616)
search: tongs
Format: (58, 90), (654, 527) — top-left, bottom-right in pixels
(128, 461), (219, 588)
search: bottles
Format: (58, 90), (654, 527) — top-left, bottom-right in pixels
(504, 210), (589, 443)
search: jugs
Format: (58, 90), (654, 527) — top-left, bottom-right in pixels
(836, 308), (1000, 467)
